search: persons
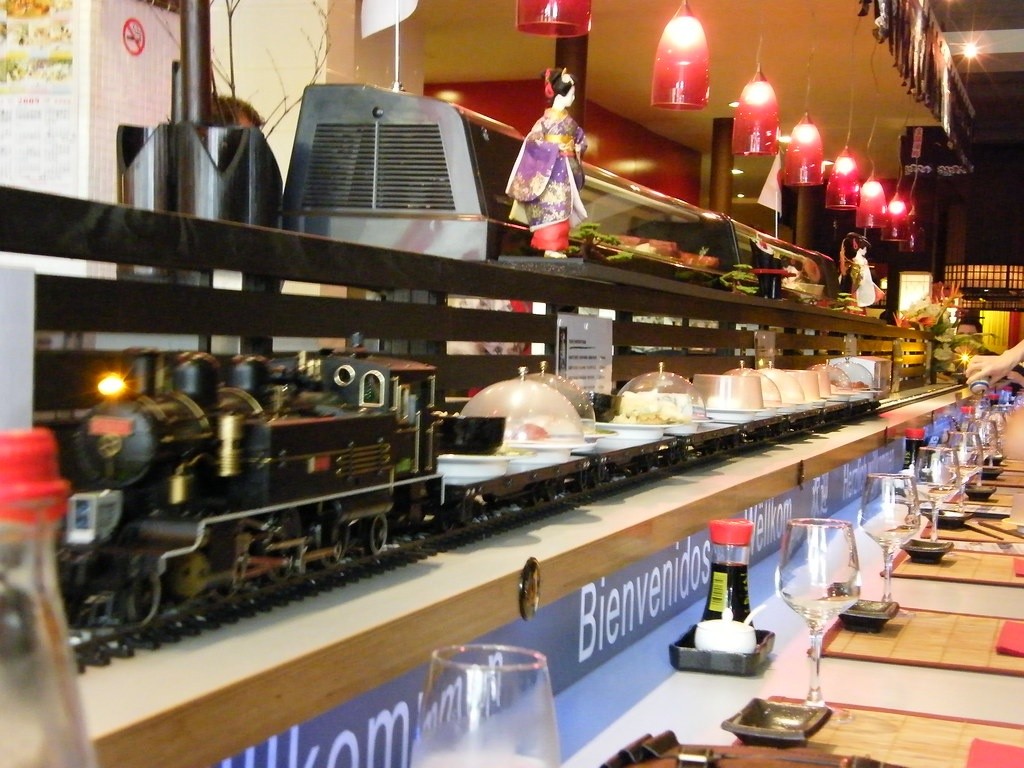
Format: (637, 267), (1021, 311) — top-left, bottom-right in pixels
(845, 231), (884, 308)
(966, 339), (1024, 388)
(501, 67), (591, 264)
(955, 315), (1024, 396)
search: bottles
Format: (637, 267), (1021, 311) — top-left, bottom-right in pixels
(0, 427), (98, 768)
(902, 428), (926, 471)
(986, 394), (999, 407)
(700, 518), (754, 629)
(960, 406), (975, 433)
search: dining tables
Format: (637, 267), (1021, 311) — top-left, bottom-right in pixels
(559, 408), (1024, 768)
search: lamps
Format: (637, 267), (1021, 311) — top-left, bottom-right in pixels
(650, 1), (714, 112)
(516, 1), (595, 38)
(730, 64), (780, 156)
(853, 173), (888, 229)
(824, 145), (862, 210)
(880, 192), (911, 242)
(898, 206), (926, 254)
(784, 112), (825, 186)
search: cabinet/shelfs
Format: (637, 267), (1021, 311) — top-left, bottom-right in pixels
(728, 216), (841, 310)
(282, 84), (742, 292)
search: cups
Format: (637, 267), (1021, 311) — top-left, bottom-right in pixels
(694, 621), (756, 654)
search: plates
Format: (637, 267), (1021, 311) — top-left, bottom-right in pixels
(661, 416), (714, 436)
(573, 430), (618, 453)
(758, 390), (884, 417)
(706, 407), (768, 423)
(595, 422), (682, 440)
(436, 454), (517, 478)
(501, 441), (596, 465)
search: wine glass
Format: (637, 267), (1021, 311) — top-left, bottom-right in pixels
(980, 411), (1007, 466)
(411, 643), (561, 768)
(945, 432), (984, 525)
(913, 447), (961, 558)
(773, 517), (861, 723)
(990, 404), (1013, 421)
(966, 419), (999, 486)
(858, 472), (921, 618)
